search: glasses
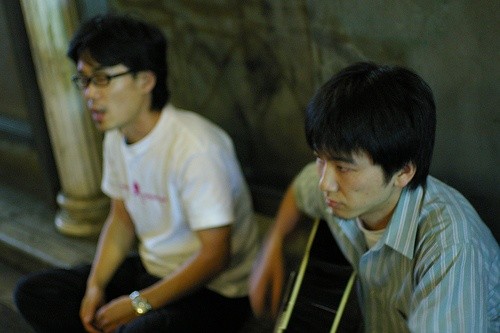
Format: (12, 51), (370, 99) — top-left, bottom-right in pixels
(71, 69), (134, 91)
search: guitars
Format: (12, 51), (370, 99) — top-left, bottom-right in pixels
(275, 214), (355, 333)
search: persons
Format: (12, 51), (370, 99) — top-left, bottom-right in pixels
(249, 58), (500, 333)
(14, 13), (262, 332)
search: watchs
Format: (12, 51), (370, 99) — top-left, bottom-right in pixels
(130, 291), (153, 317)
